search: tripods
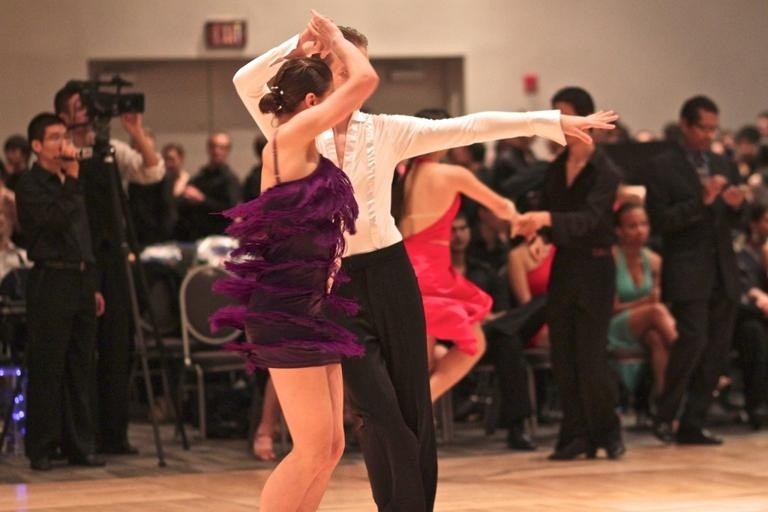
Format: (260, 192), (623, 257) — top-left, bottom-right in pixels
(93, 135), (192, 468)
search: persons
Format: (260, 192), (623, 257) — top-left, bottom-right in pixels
(231, 12), (621, 512)
(208, 7), (380, 512)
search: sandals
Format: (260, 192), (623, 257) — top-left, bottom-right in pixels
(252, 422), (278, 461)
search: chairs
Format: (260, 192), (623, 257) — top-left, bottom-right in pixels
(434, 350), (649, 447)
(0, 298), (25, 453)
(130, 258), (291, 468)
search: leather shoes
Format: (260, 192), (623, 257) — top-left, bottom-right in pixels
(60, 437), (139, 467)
(651, 414), (723, 445)
(548, 436), (625, 460)
(30, 453), (53, 469)
(507, 432), (538, 452)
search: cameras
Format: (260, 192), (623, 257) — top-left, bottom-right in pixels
(53, 146), (95, 162)
(80, 77), (146, 122)
